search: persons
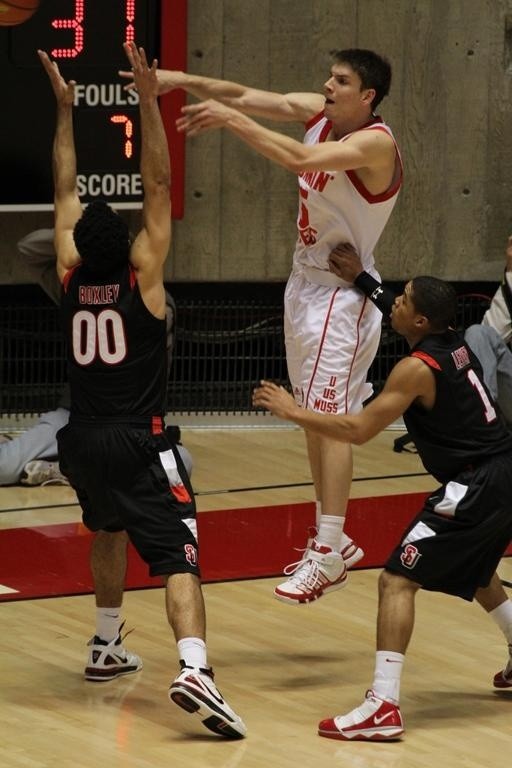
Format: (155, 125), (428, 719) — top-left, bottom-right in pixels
(36, 42), (246, 742)
(252, 242), (512, 740)
(0, 227), (194, 487)
(462, 234), (512, 432)
(117, 46), (405, 607)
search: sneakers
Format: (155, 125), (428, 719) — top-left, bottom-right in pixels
(493, 644), (512, 688)
(271, 537), (349, 607)
(299, 523), (365, 570)
(20, 459), (70, 486)
(317, 689), (407, 741)
(84, 618), (144, 682)
(165, 657), (248, 740)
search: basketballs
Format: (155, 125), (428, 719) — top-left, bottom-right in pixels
(0, 0), (36, 26)
